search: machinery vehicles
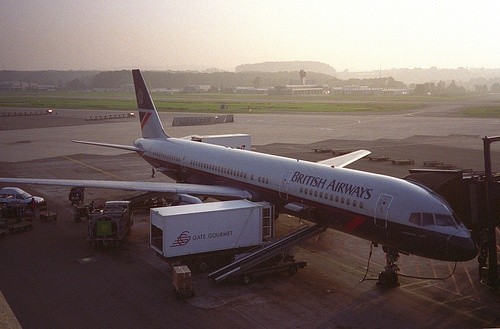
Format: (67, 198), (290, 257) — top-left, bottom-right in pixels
(85, 200), (133, 249)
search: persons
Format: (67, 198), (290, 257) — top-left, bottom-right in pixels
(478, 250), (488, 281)
(29, 197), (39, 218)
(89, 201), (95, 215)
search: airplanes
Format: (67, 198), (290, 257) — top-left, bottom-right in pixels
(0, 69), (478, 284)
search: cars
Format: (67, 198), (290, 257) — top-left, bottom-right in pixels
(0, 186), (46, 208)
